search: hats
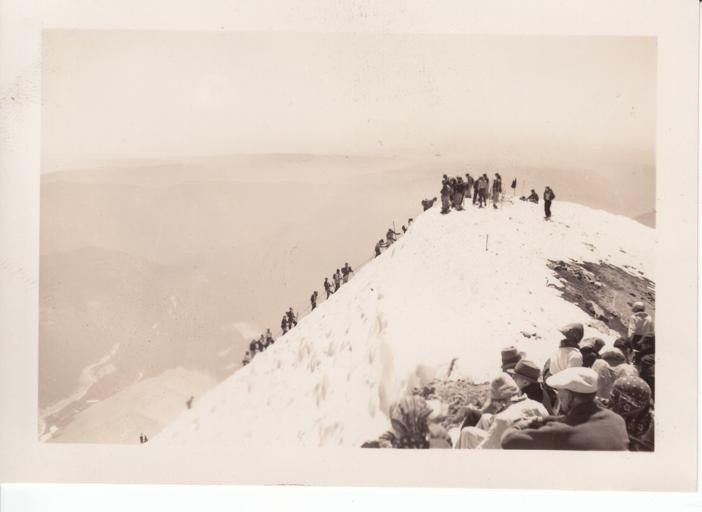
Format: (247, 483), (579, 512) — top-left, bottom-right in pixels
(545, 366), (600, 394)
(506, 360), (544, 384)
(489, 371), (520, 400)
(500, 346), (527, 370)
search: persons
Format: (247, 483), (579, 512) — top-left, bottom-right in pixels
(186, 396), (193, 410)
(139, 432), (144, 443)
(143, 435), (148, 444)
